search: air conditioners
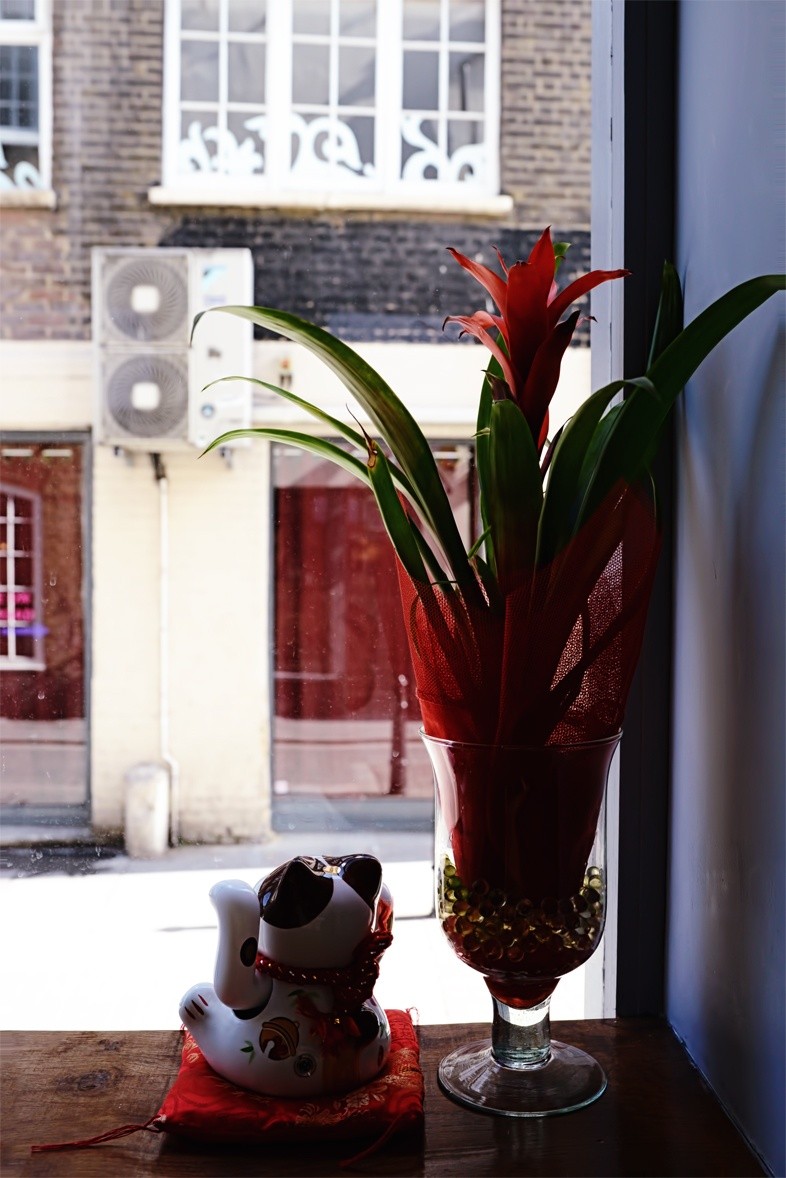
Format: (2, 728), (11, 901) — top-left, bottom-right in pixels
(90, 246), (253, 481)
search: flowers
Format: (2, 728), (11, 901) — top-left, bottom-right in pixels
(189, 225), (785, 746)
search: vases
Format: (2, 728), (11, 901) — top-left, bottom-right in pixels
(437, 727), (626, 1119)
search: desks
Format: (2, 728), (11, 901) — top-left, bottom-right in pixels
(1, 1020), (773, 1178)
